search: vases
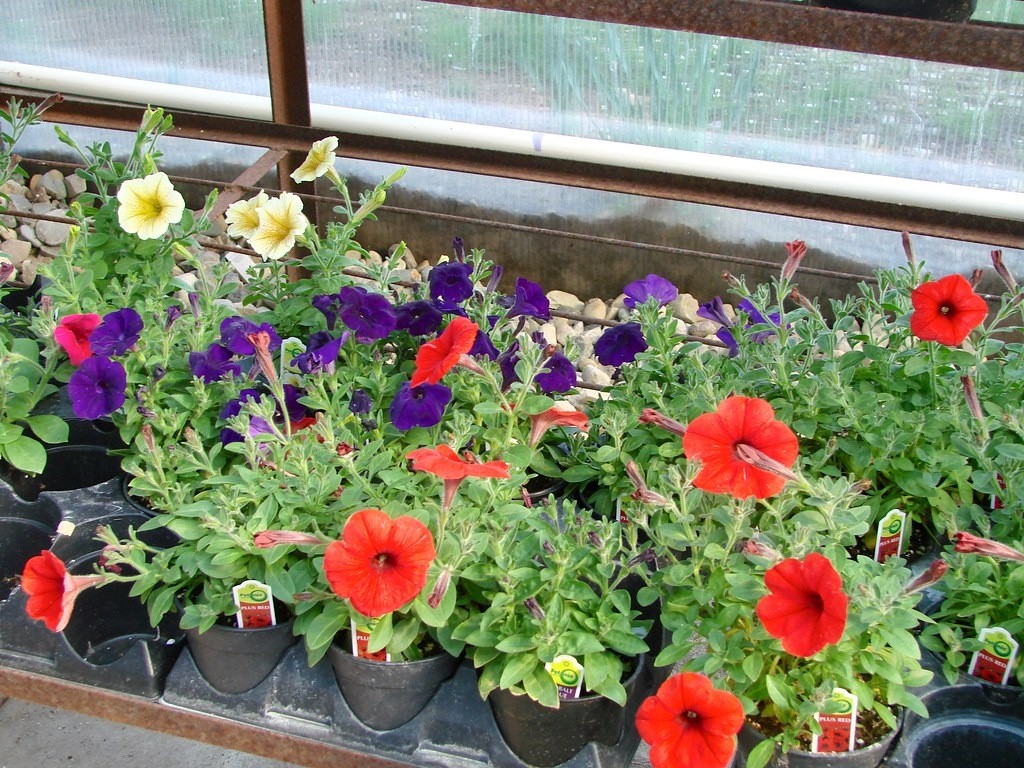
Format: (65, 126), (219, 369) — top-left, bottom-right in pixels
(0, 483), (69, 668)
(469, 651), (646, 768)
(736, 688), (904, 768)
(921, 594), (1024, 707)
(326, 628), (463, 732)
(47, 515), (185, 702)
(9, 443), (123, 506)
(887, 682), (1024, 768)
(179, 591), (300, 696)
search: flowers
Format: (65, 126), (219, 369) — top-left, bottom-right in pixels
(0, 93), (1024, 768)
